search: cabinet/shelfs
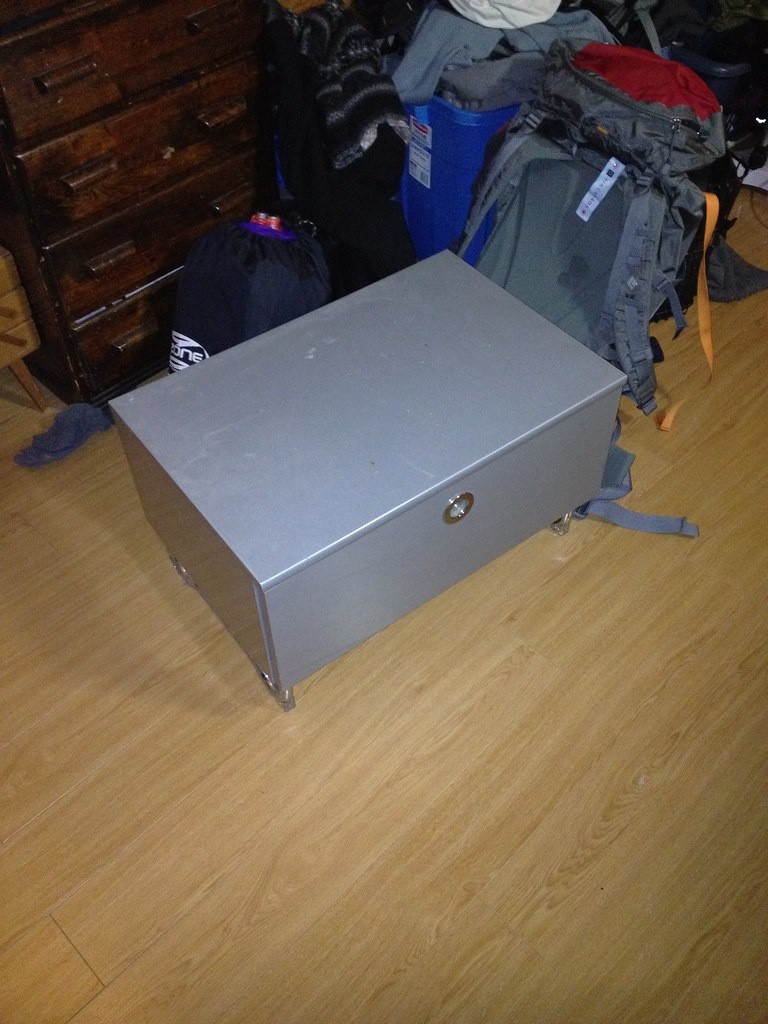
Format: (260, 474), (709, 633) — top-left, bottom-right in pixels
(0, 0), (273, 408)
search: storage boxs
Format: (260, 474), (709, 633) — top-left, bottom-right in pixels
(634, 18), (751, 106)
(393, 90), (523, 261)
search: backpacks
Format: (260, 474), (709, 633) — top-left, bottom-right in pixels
(456, 105), (705, 517)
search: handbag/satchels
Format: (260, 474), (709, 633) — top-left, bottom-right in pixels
(166, 212), (331, 375)
(533, 35), (725, 177)
